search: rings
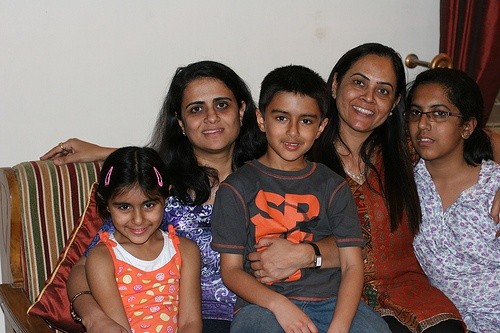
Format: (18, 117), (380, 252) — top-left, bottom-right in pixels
(59, 143), (65, 150)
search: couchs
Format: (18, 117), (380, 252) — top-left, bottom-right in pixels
(1, 159), (104, 333)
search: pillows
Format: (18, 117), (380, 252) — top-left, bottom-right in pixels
(27, 182), (104, 333)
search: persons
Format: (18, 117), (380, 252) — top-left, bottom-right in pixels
(85, 146), (203, 333)
(65, 61), (368, 333)
(38, 43), (499, 333)
(405, 67), (500, 333)
(210, 64), (394, 333)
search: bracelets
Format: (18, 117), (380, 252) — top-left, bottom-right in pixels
(69, 291), (92, 325)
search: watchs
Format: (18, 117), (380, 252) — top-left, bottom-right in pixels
(307, 243), (323, 270)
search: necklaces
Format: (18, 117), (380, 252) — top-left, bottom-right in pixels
(344, 167), (364, 182)
